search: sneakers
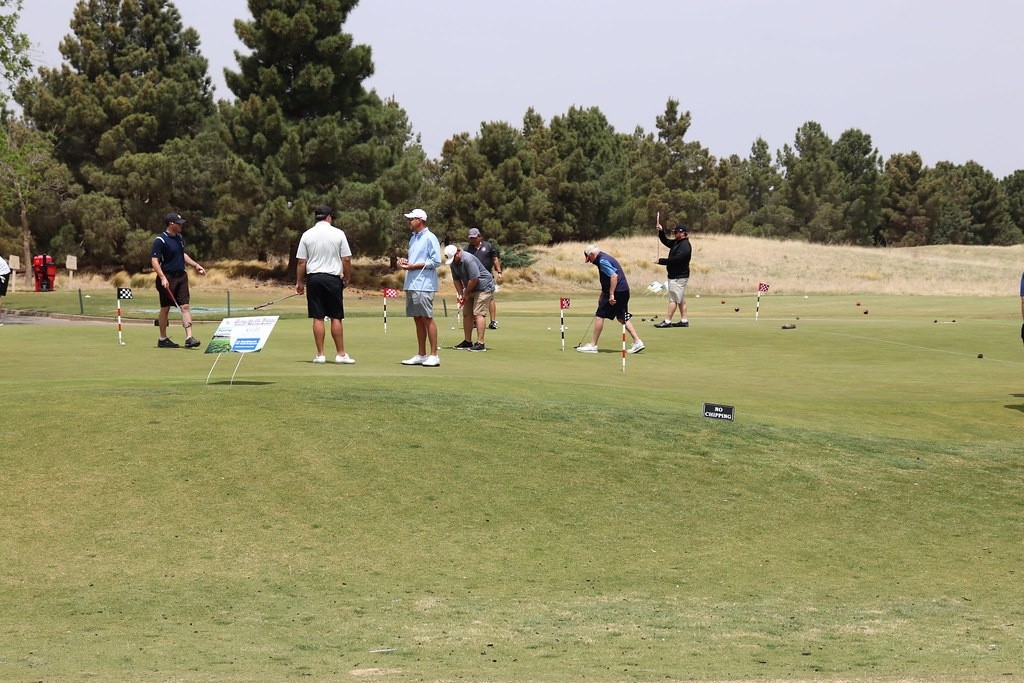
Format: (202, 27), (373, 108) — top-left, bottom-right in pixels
(672, 321), (689, 327)
(401, 354), (427, 364)
(335, 353), (355, 364)
(468, 341), (486, 352)
(577, 343), (598, 354)
(627, 339), (645, 354)
(654, 320), (671, 328)
(422, 355), (440, 366)
(313, 355), (325, 363)
(453, 340), (473, 349)
(185, 336), (200, 347)
(158, 338), (179, 348)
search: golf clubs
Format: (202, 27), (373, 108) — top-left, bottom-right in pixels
(247, 291), (306, 311)
(437, 301), (465, 351)
(166, 285), (192, 328)
(573, 306), (599, 348)
(653, 211), (660, 264)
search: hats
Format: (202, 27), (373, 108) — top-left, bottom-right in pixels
(671, 224), (687, 232)
(404, 209), (428, 221)
(315, 206), (336, 219)
(444, 245), (457, 265)
(468, 228), (479, 239)
(165, 212), (187, 226)
(584, 244), (597, 263)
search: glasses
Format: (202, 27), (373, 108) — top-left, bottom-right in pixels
(674, 231), (677, 234)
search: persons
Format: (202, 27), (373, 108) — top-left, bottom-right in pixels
(462, 227), (503, 329)
(577, 246), (646, 354)
(444, 244), (494, 352)
(395, 208), (441, 367)
(149, 213), (206, 349)
(653, 223), (692, 327)
(1020, 271), (1024, 342)
(0, 256), (10, 296)
(295, 204), (356, 364)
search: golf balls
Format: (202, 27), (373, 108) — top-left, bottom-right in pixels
(565, 327), (568, 330)
(121, 342), (126, 346)
(548, 327), (551, 330)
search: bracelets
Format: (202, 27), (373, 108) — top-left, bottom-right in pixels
(498, 271), (502, 274)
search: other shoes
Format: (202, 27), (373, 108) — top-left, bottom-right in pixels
(489, 320), (498, 329)
(473, 320), (476, 327)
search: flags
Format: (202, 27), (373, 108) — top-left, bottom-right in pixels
(385, 287), (397, 298)
(623, 311), (633, 324)
(562, 298), (570, 309)
(117, 287), (133, 299)
(758, 283), (769, 292)
